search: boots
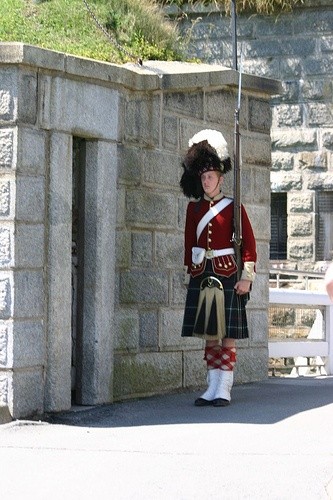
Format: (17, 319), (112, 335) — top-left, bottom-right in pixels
(194, 369), (233, 406)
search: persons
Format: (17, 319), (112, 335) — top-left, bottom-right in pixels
(324, 261), (333, 303)
(181, 154), (257, 407)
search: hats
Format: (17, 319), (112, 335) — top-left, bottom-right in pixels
(179, 129), (232, 200)
